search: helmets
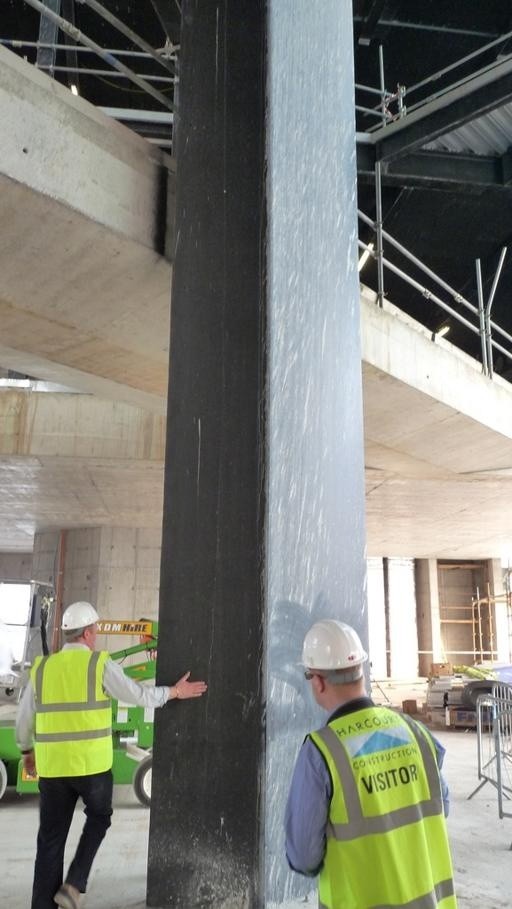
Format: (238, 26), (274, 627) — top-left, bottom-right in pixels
(293, 617), (369, 674)
(59, 598), (102, 631)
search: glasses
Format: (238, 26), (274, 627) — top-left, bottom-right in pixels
(304, 672), (329, 681)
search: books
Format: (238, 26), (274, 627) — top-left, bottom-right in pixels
(424, 677), (463, 709)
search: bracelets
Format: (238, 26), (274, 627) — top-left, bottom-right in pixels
(173, 684), (179, 698)
(20, 747), (34, 755)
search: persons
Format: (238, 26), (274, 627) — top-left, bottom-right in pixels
(14, 600), (210, 909)
(281, 618), (460, 909)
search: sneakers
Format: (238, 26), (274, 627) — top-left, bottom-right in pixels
(49, 886), (82, 908)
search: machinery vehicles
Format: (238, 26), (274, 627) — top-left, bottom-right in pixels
(0, 612), (158, 808)
